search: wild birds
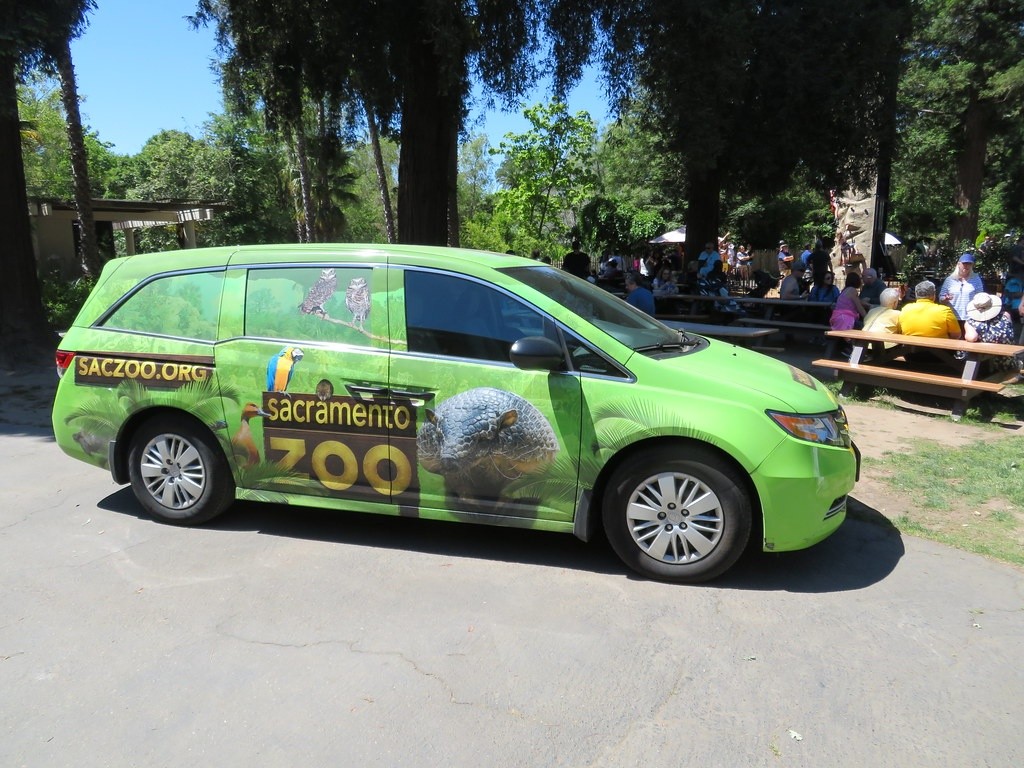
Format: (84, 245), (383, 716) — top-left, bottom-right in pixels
(230, 403), (272, 467)
(267, 347), (304, 391)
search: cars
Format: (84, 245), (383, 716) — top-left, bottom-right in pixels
(52, 241), (863, 586)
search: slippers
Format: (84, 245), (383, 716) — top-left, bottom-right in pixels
(1008, 374), (1024, 384)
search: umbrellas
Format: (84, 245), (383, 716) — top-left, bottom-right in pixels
(649, 225), (723, 244)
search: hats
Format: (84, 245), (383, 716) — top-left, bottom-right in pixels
(958, 254), (975, 263)
(915, 281), (936, 296)
(966, 292), (1002, 321)
(572, 241), (580, 248)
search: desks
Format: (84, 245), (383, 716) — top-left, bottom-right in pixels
(825, 328), (1024, 423)
(655, 319), (780, 346)
(613, 291), (836, 307)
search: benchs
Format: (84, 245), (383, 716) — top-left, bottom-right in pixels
(812, 351), (1024, 403)
(743, 344), (786, 355)
(652, 311), (835, 331)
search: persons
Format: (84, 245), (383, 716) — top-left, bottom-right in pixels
(506, 235), (1024, 384)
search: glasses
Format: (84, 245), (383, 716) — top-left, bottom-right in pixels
(826, 277), (833, 280)
